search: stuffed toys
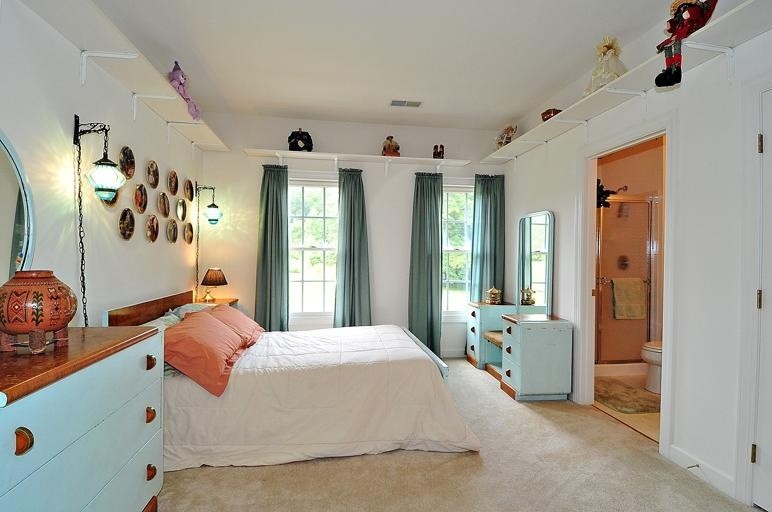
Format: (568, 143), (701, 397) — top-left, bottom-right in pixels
(167, 61), (200, 120)
(287, 127), (314, 153)
(439, 144), (444, 159)
(584, 35), (629, 90)
(493, 124), (517, 150)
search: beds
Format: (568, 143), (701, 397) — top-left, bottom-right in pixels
(101, 288), (482, 473)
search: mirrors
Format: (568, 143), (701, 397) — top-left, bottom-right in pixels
(515, 209), (555, 316)
(0, 127), (37, 289)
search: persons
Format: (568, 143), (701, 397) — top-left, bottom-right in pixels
(655, 0), (718, 87)
(382, 133), (401, 156)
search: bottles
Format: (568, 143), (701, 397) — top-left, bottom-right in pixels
(15, 239), (24, 272)
(439, 144), (445, 158)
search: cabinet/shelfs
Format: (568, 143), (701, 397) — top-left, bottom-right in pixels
(466, 302), (518, 369)
(483, 331), (503, 382)
(0, 326), (165, 512)
(193, 298), (240, 311)
(500, 314), (572, 402)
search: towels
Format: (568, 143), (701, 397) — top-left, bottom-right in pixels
(609, 277), (648, 321)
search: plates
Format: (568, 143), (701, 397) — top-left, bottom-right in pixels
(100, 145), (194, 245)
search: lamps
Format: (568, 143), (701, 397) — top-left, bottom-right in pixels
(199, 268), (228, 302)
(195, 186), (224, 287)
(73, 114), (129, 328)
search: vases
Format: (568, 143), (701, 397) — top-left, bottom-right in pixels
(0, 271), (77, 355)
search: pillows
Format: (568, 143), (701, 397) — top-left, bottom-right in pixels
(140, 308), (184, 332)
(168, 302), (217, 320)
(164, 309), (248, 398)
(206, 303), (267, 347)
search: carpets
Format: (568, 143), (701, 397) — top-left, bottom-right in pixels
(593, 374), (660, 415)
